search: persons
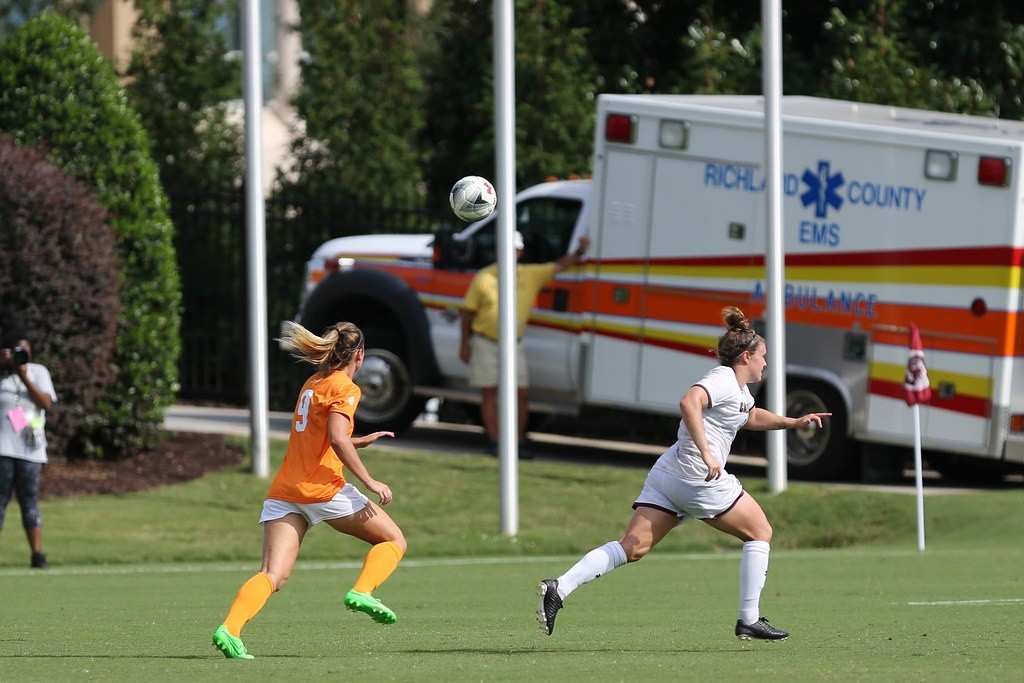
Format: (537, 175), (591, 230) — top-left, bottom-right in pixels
(213, 320), (409, 659)
(535, 305), (832, 642)
(1, 336), (57, 568)
(459, 230), (589, 460)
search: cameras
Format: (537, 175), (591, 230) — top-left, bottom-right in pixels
(11, 346), (28, 365)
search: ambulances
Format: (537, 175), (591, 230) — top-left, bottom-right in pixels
(292, 97), (1023, 484)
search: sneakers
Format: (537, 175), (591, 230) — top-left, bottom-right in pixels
(536, 579), (563, 636)
(212, 624), (256, 661)
(735, 617), (789, 643)
(344, 589), (396, 624)
(31, 551), (48, 568)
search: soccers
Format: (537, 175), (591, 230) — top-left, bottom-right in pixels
(449, 176), (497, 222)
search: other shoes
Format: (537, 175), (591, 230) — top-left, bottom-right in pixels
(519, 439), (533, 461)
(489, 441), (498, 454)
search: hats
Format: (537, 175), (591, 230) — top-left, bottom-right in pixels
(515, 231), (523, 252)
